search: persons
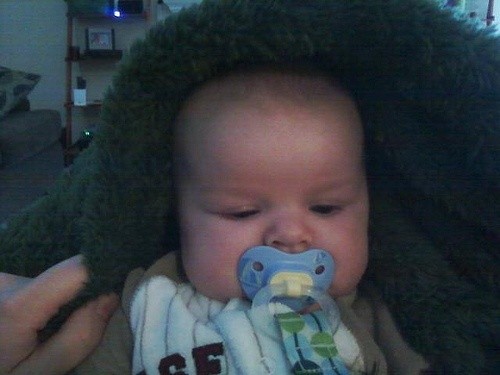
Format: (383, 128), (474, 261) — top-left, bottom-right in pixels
(0, 253), (120, 375)
(69, 62), (431, 375)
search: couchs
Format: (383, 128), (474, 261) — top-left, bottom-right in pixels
(0, 98), (67, 223)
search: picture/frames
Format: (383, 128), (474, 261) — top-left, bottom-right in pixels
(86, 27), (115, 52)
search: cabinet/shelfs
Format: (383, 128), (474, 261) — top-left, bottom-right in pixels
(65, 0), (148, 168)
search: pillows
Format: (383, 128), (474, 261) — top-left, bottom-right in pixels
(0, 66), (42, 118)
